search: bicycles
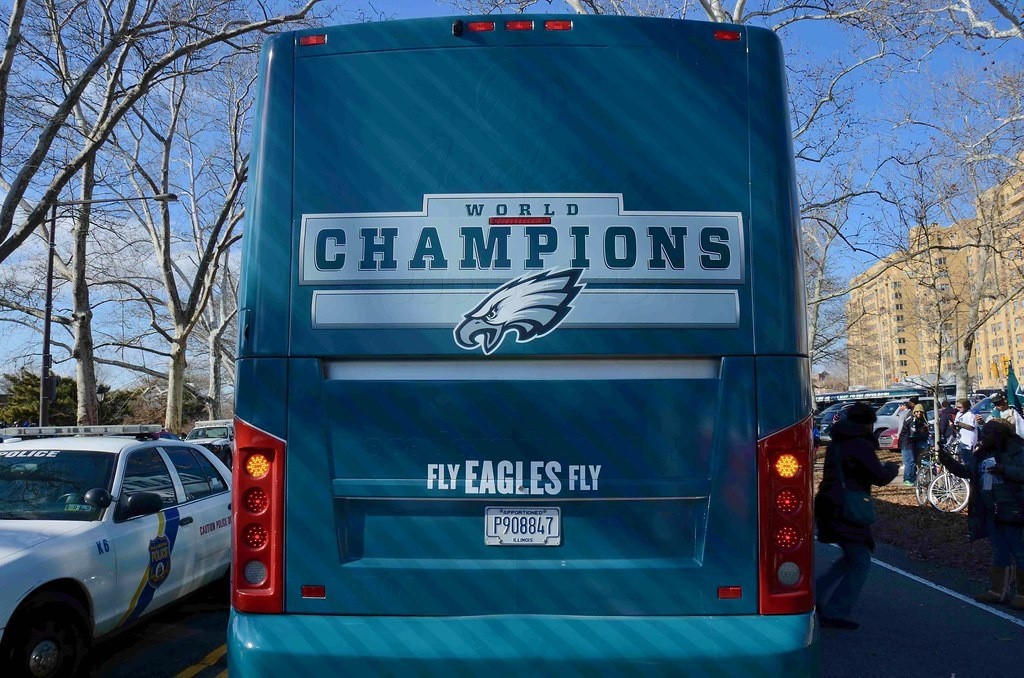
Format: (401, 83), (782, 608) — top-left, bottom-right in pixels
(915, 433), (970, 516)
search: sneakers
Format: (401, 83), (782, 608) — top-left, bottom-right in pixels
(820, 614), (860, 630)
(904, 479), (915, 488)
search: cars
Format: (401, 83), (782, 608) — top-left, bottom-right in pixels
(818, 385), (1024, 469)
(1, 418), (236, 678)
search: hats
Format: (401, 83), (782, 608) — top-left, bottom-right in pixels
(845, 402), (876, 426)
(991, 391), (1007, 403)
(913, 403), (925, 418)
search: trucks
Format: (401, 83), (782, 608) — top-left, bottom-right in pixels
(181, 419), (234, 472)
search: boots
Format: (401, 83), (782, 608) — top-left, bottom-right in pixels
(1009, 566), (1024, 610)
(981, 565), (1012, 603)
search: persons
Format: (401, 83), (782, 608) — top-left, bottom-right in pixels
(812, 395), (1024, 488)
(813, 399), (901, 630)
(937, 419), (1024, 612)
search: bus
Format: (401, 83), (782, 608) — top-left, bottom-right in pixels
(811, 386), (946, 447)
(222, 10), (827, 678)
